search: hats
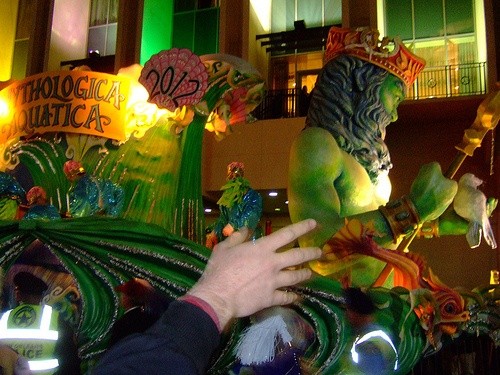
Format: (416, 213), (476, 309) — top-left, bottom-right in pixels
(13, 272), (48, 295)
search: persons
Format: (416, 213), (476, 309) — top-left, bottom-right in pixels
(0, 218), (399, 375)
(288, 26), (500, 290)
